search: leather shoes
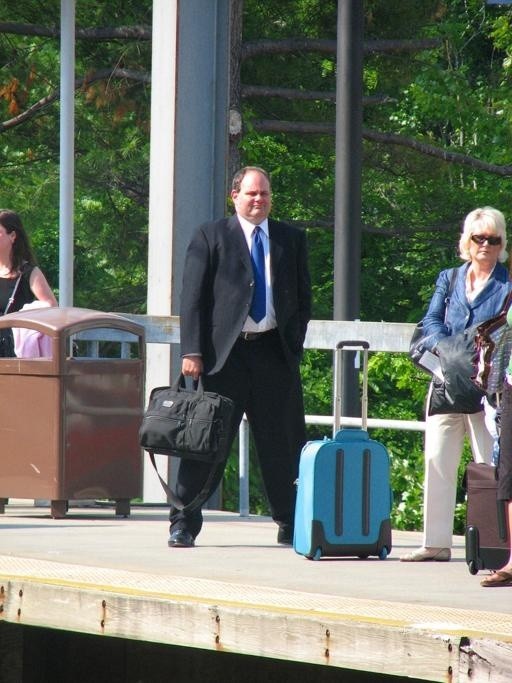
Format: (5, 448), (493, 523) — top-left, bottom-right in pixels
(277, 526), (292, 543)
(167, 529), (195, 547)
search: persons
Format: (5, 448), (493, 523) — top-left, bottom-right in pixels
(480, 294), (512, 587)
(0, 208), (60, 504)
(399, 205), (512, 560)
(166, 165), (312, 548)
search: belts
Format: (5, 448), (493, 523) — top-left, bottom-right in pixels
(238, 326), (280, 342)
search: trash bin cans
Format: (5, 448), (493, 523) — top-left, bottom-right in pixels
(0, 306), (147, 519)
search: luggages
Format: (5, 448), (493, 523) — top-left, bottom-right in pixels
(461, 455), (510, 573)
(288, 341), (394, 560)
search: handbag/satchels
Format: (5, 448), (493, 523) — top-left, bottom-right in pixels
(437, 318), (488, 413)
(407, 265), (452, 378)
(136, 369), (242, 467)
(468, 286), (511, 399)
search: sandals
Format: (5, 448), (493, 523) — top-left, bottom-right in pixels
(399, 546), (451, 561)
(479, 569), (511, 587)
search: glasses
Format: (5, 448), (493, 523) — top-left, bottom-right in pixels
(469, 236), (503, 247)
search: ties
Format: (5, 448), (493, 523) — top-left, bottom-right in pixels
(246, 226), (266, 325)
